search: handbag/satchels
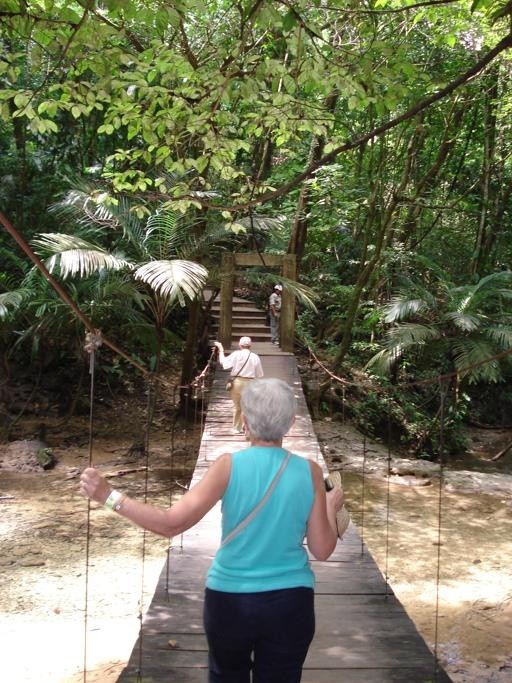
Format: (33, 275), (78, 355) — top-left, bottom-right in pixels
(225, 380), (233, 391)
(327, 470), (351, 541)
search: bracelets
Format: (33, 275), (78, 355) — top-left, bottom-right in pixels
(105, 490), (122, 509)
(113, 496), (127, 511)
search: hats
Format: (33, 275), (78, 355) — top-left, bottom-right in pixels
(239, 335), (252, 346)
(274, 284), (282, 291)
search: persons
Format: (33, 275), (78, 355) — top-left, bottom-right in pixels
(213, 336), (264, 435)
(269, 284), (282, 345)
(80, 377), (350, 683)
